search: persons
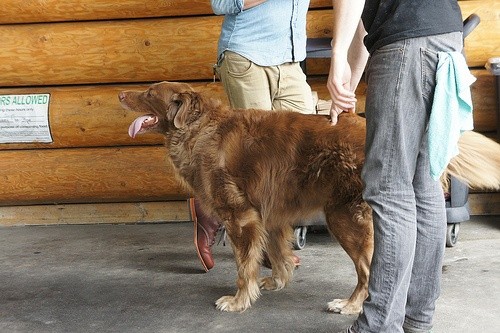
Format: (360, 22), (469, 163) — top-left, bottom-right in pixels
(187, 0), (317, 272)
(326, 0), (463, 333)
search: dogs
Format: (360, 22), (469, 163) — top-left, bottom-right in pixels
(118, 81), (500, 315)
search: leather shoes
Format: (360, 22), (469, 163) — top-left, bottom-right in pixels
(187, 196), (220, 272)
(262, 251), (299, 268)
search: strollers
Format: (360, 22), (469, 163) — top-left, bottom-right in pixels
(293, 12), (480, 251)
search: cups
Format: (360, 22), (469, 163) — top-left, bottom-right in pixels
(485, 57), (500, 75)
